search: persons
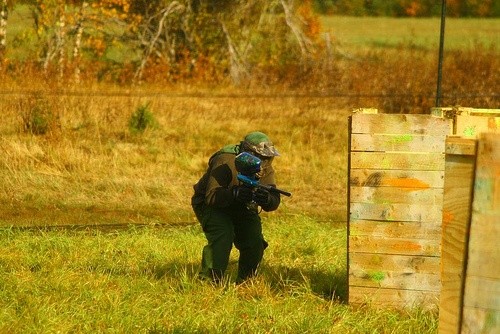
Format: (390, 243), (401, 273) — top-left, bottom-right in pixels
(190, 132), (280, 283)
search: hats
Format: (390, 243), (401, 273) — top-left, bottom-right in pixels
(243, 131), (280, 157)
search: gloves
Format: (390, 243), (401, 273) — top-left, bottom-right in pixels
(254, 188), (269, 207)
(231, 185), (253, 203)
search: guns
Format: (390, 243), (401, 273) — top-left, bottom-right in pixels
(234, 152), (291, 200)
